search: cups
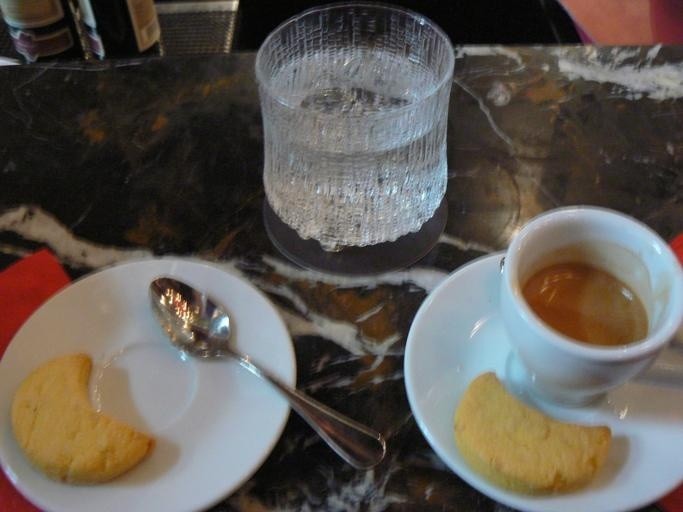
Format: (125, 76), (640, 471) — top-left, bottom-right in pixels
(250, 0), (457, 252)
(500, 205), (683, 412)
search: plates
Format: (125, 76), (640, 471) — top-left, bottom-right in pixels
(0, 257), (296, 510)
(405, 252), (683, 510)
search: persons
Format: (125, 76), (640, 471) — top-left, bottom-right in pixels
(558, 0), (683, 46)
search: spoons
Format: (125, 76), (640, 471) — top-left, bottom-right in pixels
(147, 277), (388, 472)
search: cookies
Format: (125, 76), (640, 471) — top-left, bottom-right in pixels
(453, 371), (613, 494)
(10, 352), (153, 483)
